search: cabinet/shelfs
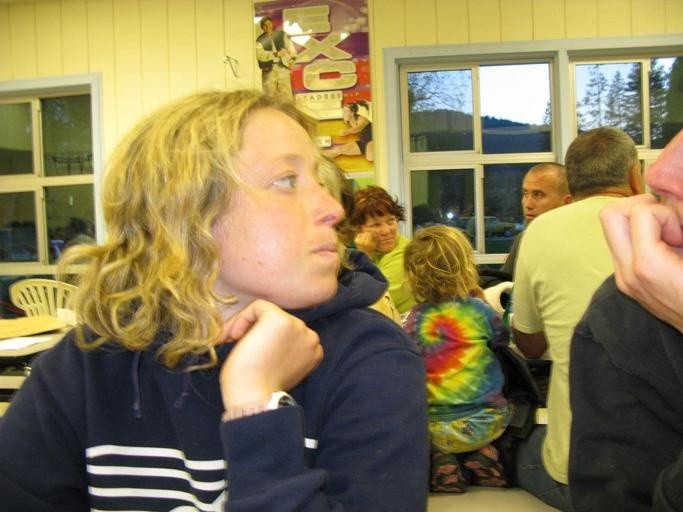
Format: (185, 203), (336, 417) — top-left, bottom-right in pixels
(0, 333), (66, 422)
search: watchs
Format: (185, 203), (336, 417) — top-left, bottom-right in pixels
(219, 391), (298, 422)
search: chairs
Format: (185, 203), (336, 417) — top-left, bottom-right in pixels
(8, 278), (81, 321)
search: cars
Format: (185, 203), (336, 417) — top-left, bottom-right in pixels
(453, 215), (522, 237)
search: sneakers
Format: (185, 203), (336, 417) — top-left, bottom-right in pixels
(428, 448), (510, 494)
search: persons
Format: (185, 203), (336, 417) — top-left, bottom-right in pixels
(0, 216), (95, 305)
(0, 89), (431, 510)
(568, 129), (682, 512)
(323, 99), (374, 162)
(256, 16), (298, 103)
